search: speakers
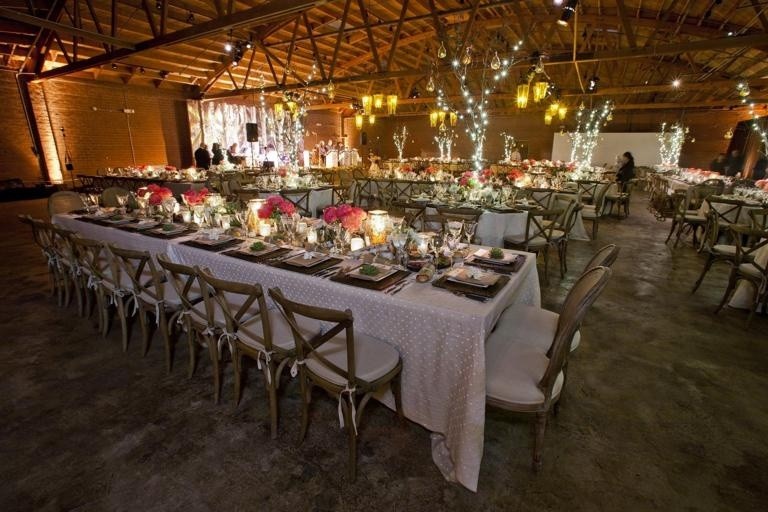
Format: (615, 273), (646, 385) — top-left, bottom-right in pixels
(362, 131), (367, 145)
(246, 123), (258, 142)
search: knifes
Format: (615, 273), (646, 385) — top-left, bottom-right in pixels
(384, 280), (407, 295)
(313, 266), (342, 280)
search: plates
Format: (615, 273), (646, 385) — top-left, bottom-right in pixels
(153, 225), (189, 236)
(194, 235), (235, 246)
(128, 220), (158, 231)
(238, 241), (280, 256)
(346, 264), (398, 283)
(283, 251), (328, 266)
(446, 267), (500, 289)
(476, 247), (517, 263)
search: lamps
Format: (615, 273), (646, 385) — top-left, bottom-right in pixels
(517, 52), (554, 111)
(558, 0), (578, 27)
(226, 31), (244, 66)
(354, 78), (457, 129)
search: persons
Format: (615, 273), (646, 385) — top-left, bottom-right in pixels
(723, 149), (744, 178)
(750, 151), (768, 182)
(195, 142), (211, 170)
(710, 152), (729, 174)
(511, 146), (523, 163)
(226, 142), (238, 162)
(211, 143), (224, 165)
(617, 149), (637, 219)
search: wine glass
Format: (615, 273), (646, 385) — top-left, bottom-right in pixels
(516, 174), (560, 189)
(430, 180), (517, 208)
(115, 191), (478, 269)
(731, 184), (767, 207)
(253, 173), (319, 193)
